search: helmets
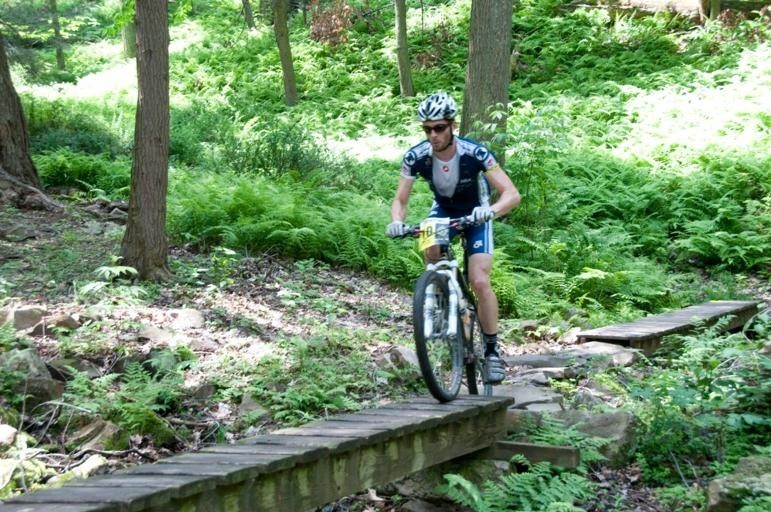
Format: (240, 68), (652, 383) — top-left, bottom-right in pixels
(417, 94), (458, 122)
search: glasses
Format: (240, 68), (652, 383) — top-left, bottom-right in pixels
(422, 123), (450, 134)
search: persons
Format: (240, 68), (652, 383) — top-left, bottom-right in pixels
(385, 89), (521, 382)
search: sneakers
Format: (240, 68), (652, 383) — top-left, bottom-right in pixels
(484, 354), (505, 384)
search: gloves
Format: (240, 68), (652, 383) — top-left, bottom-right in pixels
(387, 221), (409, 238)
(471, 207), (496, 226)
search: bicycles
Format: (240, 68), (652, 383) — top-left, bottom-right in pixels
(390, 215), (498, 403)
